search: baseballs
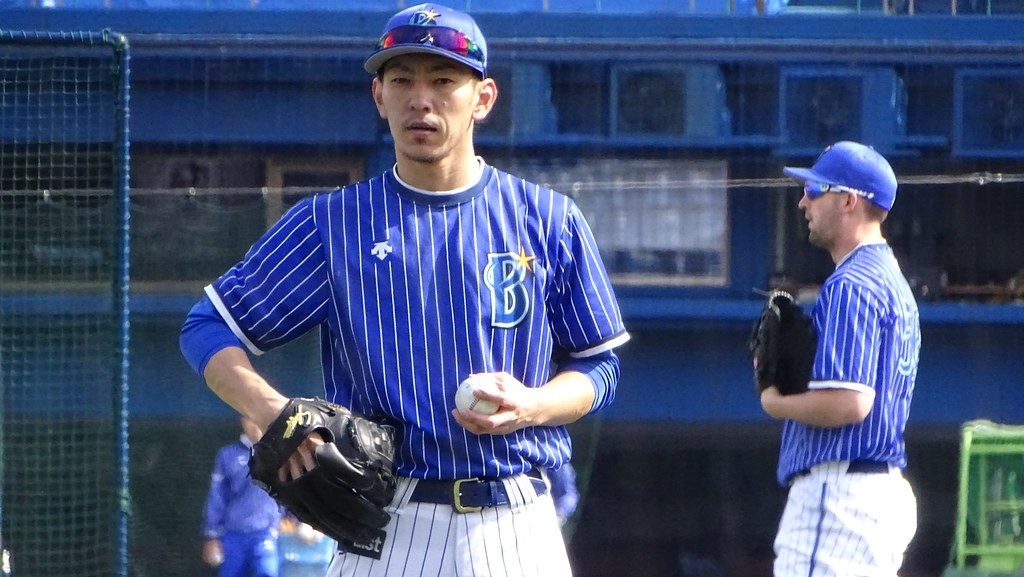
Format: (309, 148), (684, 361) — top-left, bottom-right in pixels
(453, 372), (502, 418)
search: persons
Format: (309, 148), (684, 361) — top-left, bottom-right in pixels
(749, 140), (922, 577)
(180, 3), (632, 576)
(199, 413), (287, 577)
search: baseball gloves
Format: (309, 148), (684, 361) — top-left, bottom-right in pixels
(745, 289), (820, 399)
(244, 392), (403, 550)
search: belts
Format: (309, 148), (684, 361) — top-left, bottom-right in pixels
(393, 468), (548, 515)
(798, 460), (889, 474)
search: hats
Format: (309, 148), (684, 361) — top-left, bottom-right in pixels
(783, 141), (897, 211)
(364, 3), (487, 80)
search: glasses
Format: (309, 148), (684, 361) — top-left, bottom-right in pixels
(803, 182), (874, 200)
